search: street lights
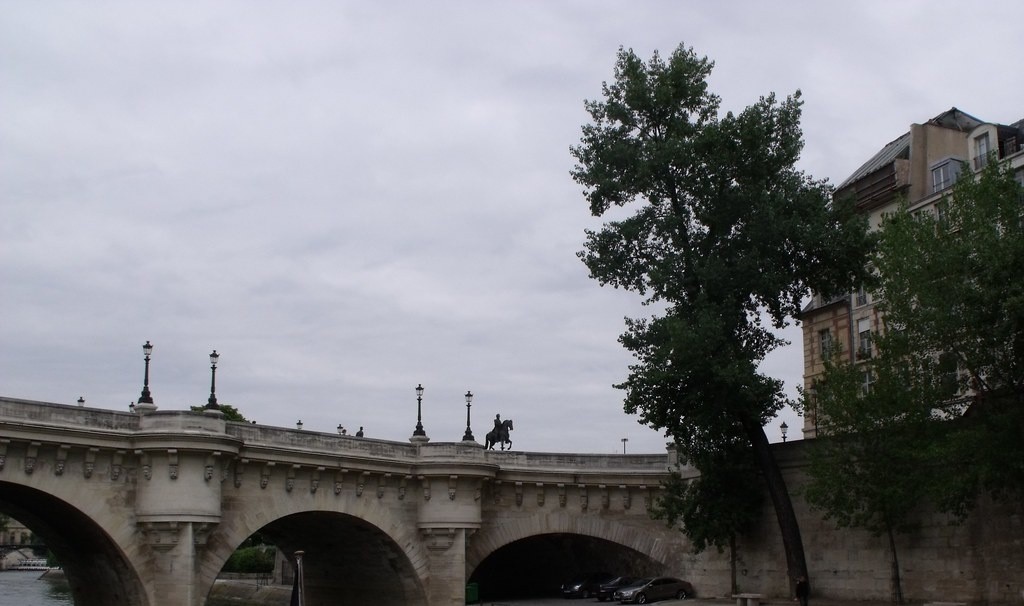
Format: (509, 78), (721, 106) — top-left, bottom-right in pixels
(621, 438), (627, 452)
(133, 341), (158, 412)
(203, 348), (226, 415)
(460, 391), (476, 443)
(409, 382), (430, 443)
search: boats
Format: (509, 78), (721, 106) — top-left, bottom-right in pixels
(18, 559), (51, 571)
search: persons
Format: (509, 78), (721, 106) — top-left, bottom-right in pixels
(341, 426), (363, 437)
(493, 414), (502, 444)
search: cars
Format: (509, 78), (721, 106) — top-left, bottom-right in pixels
(559, 572), (614, 598)
(594, 573), (643, 602)
(614, 577), (694, 605)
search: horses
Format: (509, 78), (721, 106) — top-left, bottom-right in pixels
(484, 420), (514, 450)
